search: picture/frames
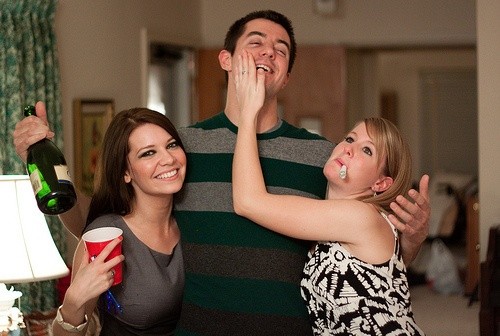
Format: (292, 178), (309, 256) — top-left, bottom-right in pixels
(72, 96), (116, 196)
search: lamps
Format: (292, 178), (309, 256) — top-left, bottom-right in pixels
(0, 175), (70, 336)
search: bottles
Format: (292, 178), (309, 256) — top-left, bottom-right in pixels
(23, 105), (77, 215)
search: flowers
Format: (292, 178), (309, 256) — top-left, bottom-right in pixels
(0, 283), (26, 331)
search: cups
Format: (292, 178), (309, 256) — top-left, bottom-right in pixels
(81, 227), (125, 287)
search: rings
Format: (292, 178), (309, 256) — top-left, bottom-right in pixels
(241, 71), (248, 75)
(109, 270), (115, 276)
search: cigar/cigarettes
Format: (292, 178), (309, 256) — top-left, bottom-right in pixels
(339, 165), (347, 180)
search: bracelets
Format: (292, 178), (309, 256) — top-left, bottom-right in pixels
(55, 305), (90, 333)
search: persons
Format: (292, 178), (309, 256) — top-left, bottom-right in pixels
(12, 9), (432, 336)
(51, 107), (187, 336)
(232, 48), (426, 336)
